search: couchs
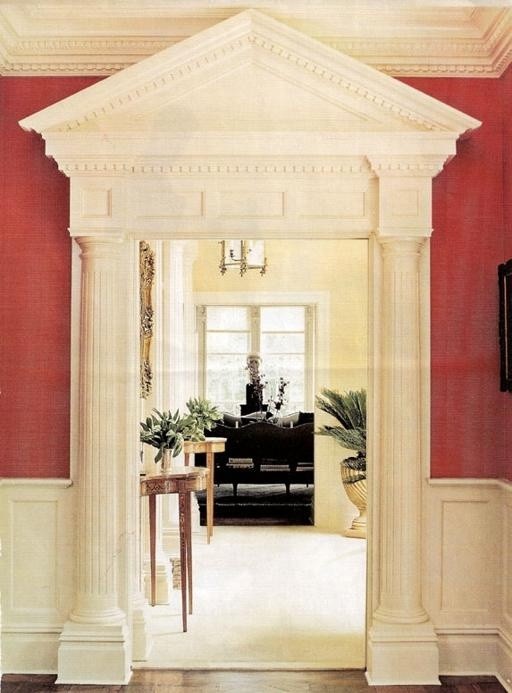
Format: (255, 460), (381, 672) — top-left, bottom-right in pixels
(201, 405), (314, 500)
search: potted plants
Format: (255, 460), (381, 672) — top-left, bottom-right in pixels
(139, 405), (195, 472)
(311, 382), (369, 538)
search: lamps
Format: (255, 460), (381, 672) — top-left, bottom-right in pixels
(218, 239), (269, 276)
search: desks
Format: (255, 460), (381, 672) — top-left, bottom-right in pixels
(180, 438), (227, 547)
(139, 458), (209, 637)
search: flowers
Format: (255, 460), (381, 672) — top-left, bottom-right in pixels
(242, 357), (269, 416)
(267, 375), (289, 417)
